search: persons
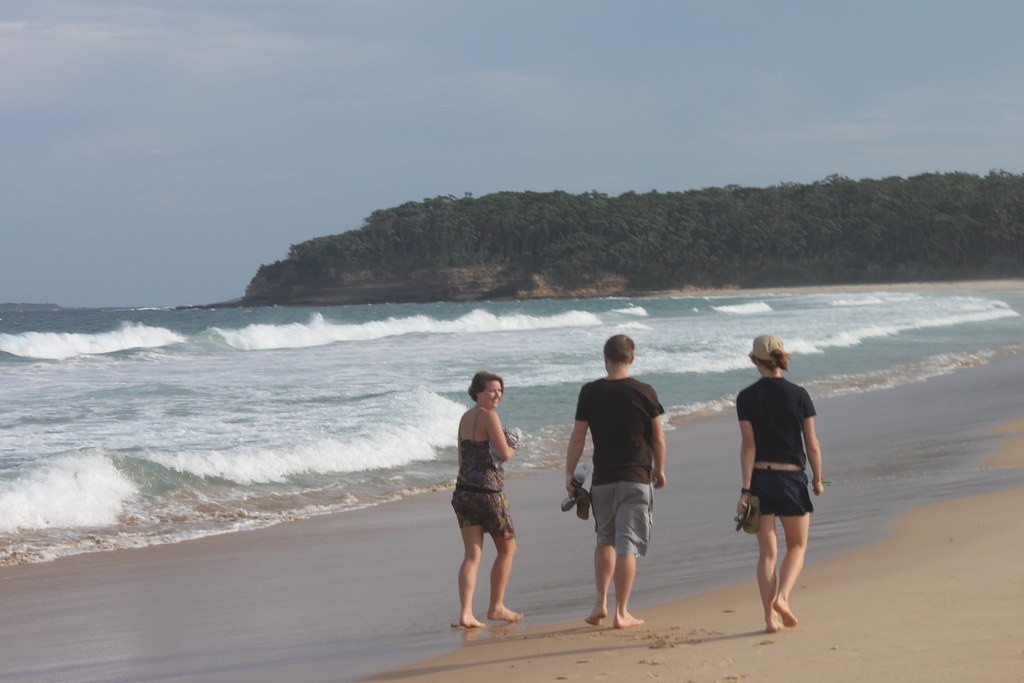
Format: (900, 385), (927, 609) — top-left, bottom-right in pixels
(737, 334), (824, 632)
(565, 333), (665, 629)
(449, 370), (524, 629)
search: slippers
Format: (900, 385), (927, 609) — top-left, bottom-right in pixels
(560, 495), (576, 511)
(575, 488), (590, 521)
(735, 503), (751, 531)
(742, 495), (761, 534)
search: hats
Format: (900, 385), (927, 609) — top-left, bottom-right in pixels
(749, 334), (784, 361)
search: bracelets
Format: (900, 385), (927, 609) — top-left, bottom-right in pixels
(741, 487), (750, 494)
